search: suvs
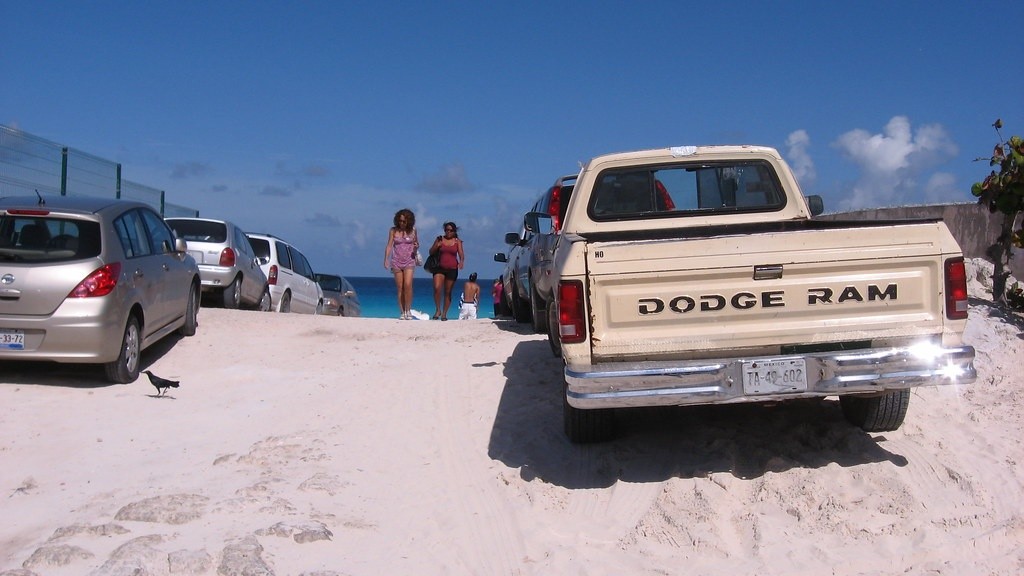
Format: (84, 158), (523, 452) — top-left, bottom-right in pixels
(243, 232), (324, 316)
(313, 273), (361, 318)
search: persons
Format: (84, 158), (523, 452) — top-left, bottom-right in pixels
(493, 274), (503, 319)
(384, 209), (419, 320)
(458, 272), (480, 320)
(429, 222), (464, 321)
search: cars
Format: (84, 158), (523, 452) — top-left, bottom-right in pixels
(1, 186), (203, 384)
(491, 170), (676, 334)
(154, 217), (273, 312)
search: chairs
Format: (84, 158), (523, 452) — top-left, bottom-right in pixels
(612, 180), (666, 214)
(48, 234), (78, 249)
(19, 224), (48, 244)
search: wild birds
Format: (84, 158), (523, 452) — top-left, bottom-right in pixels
(140, 369), (181, 398)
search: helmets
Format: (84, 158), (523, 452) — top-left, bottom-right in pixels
(469, 273), (477, 281)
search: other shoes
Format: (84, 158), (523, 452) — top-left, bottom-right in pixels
(406, 312), (412, 319)
(441, 316), (446, 321)
(433, 311), (441, 320)
(400, 312), (406, 320)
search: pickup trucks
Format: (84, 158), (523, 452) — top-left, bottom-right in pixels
(542, 145), (983, 443)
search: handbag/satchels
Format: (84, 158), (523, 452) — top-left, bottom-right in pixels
(424, 235), (442, 273)
(415, 251), (422, 266)
